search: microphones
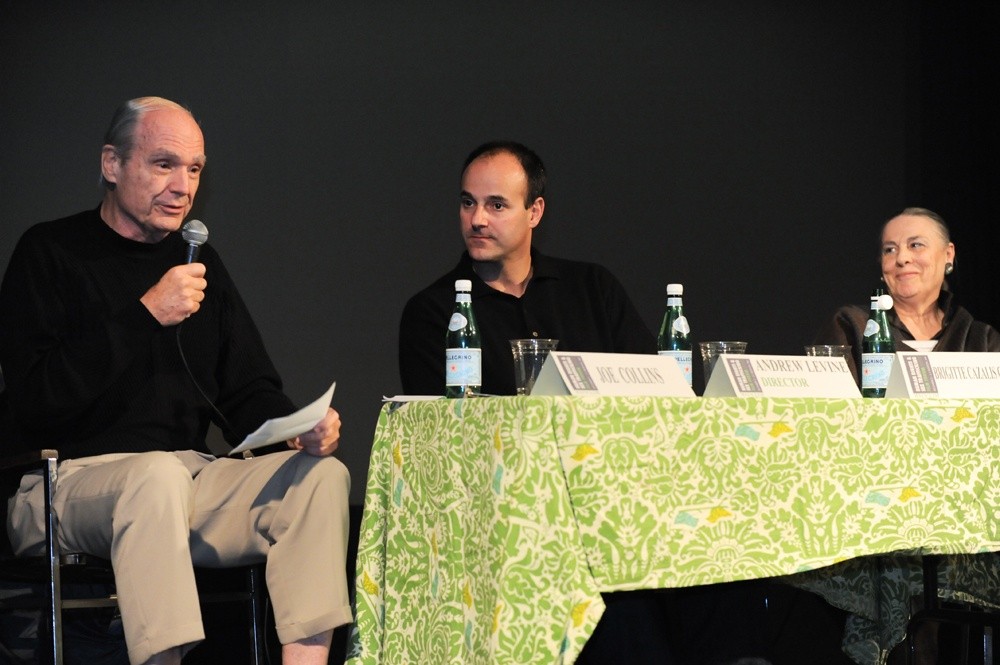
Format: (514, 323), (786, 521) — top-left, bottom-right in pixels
(178, 220), (209, 264)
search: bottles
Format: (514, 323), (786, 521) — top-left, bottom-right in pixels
(861, 288), (895, 398)
(445, 279), (482, 397)
(657, 283), (693, 391)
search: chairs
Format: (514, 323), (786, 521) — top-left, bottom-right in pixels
(10, 444), (270, 665)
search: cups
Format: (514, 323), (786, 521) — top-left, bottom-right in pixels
(698, 342), (748, 390)
(509, 339), (560, 396)
(804, 345), (851, 368)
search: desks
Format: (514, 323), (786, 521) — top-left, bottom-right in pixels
(340, 399), (999, 662)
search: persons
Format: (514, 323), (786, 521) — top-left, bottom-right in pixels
(1, 96), (356, 665)
(808, 205), (1000, 665)
(398, 140), (765, 664)
